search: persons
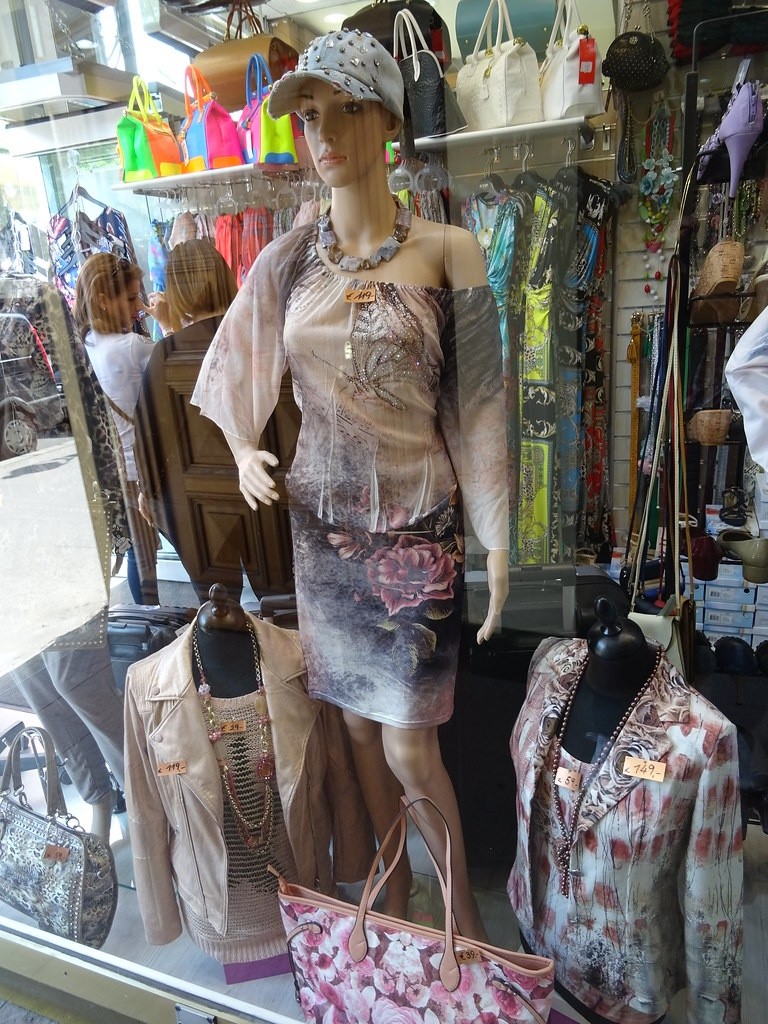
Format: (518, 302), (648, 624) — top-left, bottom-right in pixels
(185, 24), (509, 950)
(507, 597), (743, 1022)
(129, 237), (304, 609)
(0, 269), (128, 844)
(123, 583), (377, 948)
(72, 252), (180, 607)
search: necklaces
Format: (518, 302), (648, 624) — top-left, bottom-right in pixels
(191, 616), (275, 856)
(318, 194), (413, 273)
(612, 92), (768, 301)
(548, 636), (664, 898)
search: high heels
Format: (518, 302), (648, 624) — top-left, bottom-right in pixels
(697, 82), (763, 200)
(720, 486), (747, 526)
(684, 407), (732, 446)
(690, 241), (745, 326)
(735, 248), (768, 323)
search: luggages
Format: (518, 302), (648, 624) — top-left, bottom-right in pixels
(57, 603), (199, 786)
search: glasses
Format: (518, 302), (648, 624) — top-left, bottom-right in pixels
(111, 257), (130, 276)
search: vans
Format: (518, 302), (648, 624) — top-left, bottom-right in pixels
(0, 312), (66, 462)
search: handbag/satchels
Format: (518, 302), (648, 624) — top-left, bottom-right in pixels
(0, 727), (118, 951)
(116, 0), (603, 183)
(619, 542), (697, 685)
(602, 0), (669, 91)
(267, 795), (555, 1024)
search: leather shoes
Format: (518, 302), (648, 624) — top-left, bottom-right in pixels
(679, 526), (719, 582)
(717, 529), (768, 584)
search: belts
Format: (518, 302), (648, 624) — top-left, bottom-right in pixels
(625, 312), (665, 560)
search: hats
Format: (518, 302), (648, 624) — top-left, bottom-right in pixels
(266, 27), (405, 125)
